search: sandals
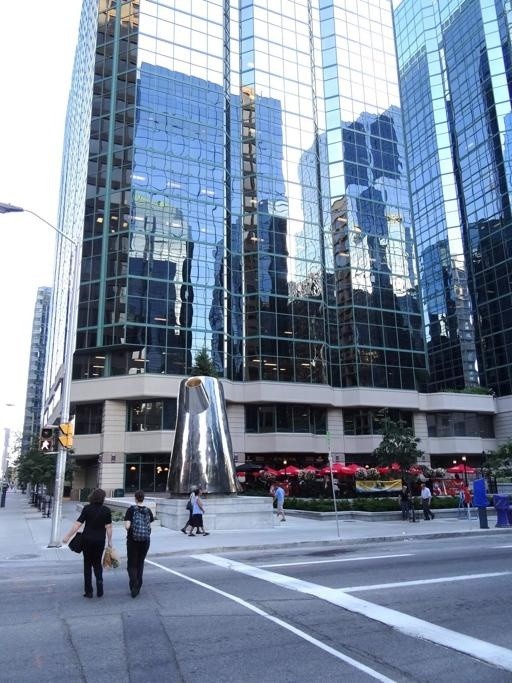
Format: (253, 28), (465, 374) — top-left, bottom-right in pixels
(181, 528), (210, 536)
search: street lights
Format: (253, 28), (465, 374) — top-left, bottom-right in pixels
(462, 456), (469, 486)
(283, 460), (287, 480)
(0, 203), (79, 547)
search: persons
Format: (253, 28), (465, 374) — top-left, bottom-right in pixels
(62, 489), (118, 598)
(270, 482), (287, 521)
(180, 488), (209, 536)
(420, 483), (435, 521)
(399, 485), (412, 521)
(124, 489), (154, 597)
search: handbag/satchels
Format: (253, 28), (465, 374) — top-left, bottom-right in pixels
(69, 533), (84, 553)
(186, 501), (193, 509)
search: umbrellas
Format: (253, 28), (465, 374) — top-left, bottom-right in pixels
(236, 461), (476, 486)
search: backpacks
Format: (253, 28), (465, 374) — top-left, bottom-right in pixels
(131, 505), (151, 543)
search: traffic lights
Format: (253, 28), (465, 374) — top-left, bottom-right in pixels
(39, 428), (53, 451)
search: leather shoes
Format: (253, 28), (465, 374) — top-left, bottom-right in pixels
(97, 591), (103, 596)
(83, 593), (93, 598)
